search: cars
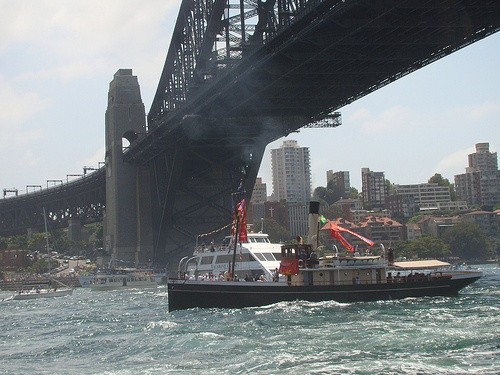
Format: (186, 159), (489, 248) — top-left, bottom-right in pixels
(62, 255), (91, 263)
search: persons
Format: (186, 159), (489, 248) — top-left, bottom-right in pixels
(386, 272), (444, 284)
(301, 250), (307, 268)
(351, 246), (382, 257)
(201, 239), (227, 253)
(310, 250), (317, 268)
(178, 267), (279, 282)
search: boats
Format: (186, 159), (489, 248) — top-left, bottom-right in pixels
(167, 224), (482, 316)
(91, 268), (157, 289)
(12, 291), (75, 298)
